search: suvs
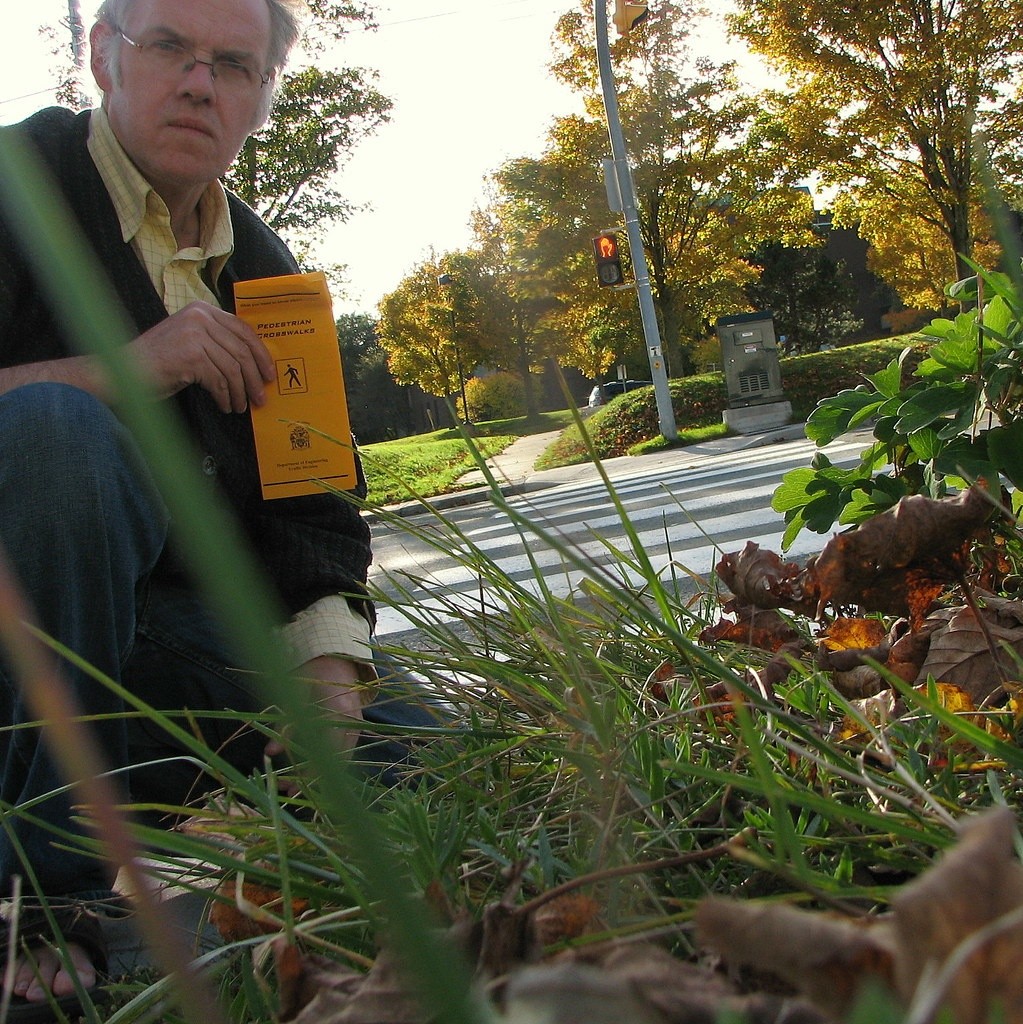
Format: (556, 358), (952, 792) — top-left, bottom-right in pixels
(588, 378), (652, 408)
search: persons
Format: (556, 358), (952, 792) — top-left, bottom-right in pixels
(0, 2), (471, 1024)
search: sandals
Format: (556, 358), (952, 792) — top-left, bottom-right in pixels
(0, 916), (112, 1022)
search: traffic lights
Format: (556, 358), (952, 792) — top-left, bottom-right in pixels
(612, 0), (651, 37)
(591, 234), (624, 289)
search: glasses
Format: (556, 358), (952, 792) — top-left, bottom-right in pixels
(114, 26), (271, 95)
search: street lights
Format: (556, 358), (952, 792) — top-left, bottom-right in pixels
(438, 274), (472, 425)
